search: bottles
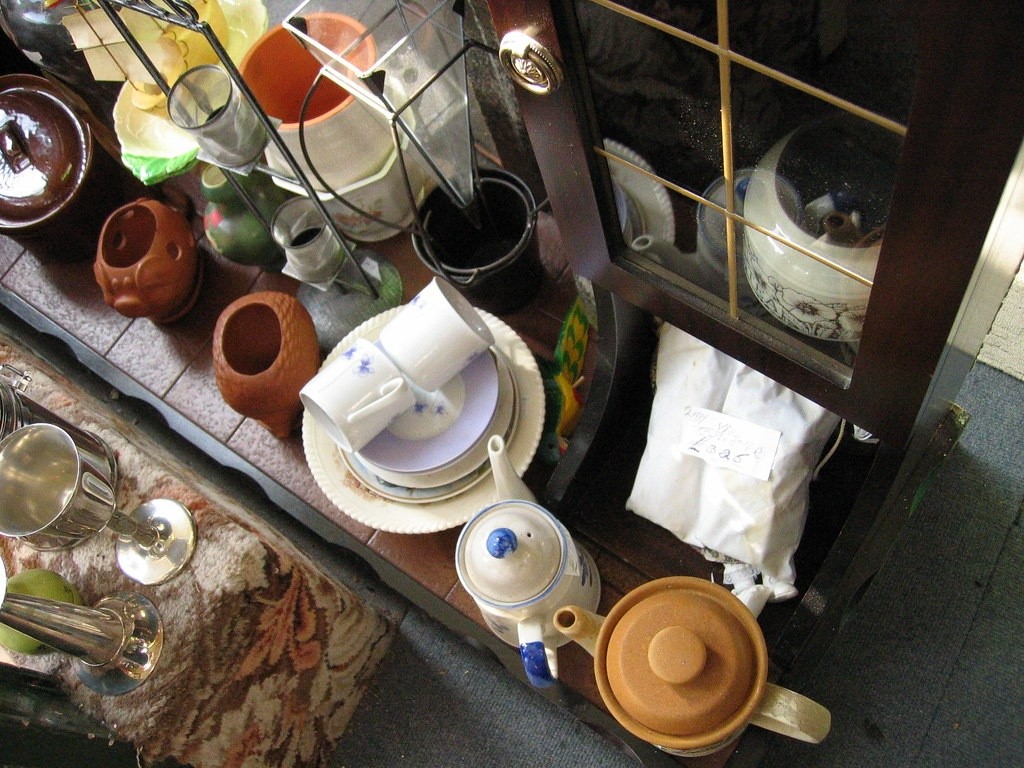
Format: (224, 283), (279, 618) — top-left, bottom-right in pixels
(200, 161), (288, 266)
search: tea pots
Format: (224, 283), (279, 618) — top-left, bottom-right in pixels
(632, 169), (803, 317)
(553, 576), (831, 756)
(454, 434), (599, 688)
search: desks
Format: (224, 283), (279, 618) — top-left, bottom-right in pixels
(0, 326), (398, 768)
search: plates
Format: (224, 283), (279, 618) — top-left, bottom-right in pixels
(112, 0), (268, 155)
(603, 138), (675, 247)
(302, 304), (544, 533)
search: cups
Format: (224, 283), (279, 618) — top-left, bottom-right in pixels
(379, 278), (495, 391)
(0, 376), (117, 491)
(272, 196), (345, 283)
(238, 14), (396, 191)
(167, 65), (266, 167)
(301, 340), (414, 454)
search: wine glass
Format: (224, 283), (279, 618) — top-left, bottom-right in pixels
(0, 424), (198, 585)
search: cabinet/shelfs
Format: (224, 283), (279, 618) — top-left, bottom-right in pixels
(0, 0), (1024, 768)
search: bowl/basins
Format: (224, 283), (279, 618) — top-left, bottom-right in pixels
(611, 184), (633, 242)
